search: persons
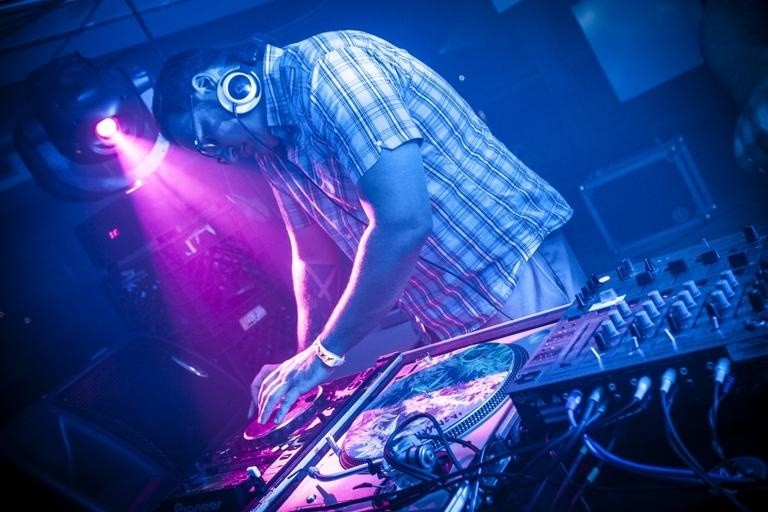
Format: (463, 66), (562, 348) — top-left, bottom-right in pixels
(152, 28), (587, 425)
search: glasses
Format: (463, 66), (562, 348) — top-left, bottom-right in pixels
(190, 96), (220, 157)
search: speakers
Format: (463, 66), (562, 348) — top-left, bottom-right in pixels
(0, 329), (251, 512)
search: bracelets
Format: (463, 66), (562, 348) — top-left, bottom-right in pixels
(312, 336), (346, 368)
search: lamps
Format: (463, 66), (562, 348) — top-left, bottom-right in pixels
(30, 50), (145, 166)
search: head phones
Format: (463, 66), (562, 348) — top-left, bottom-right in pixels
(216, 34), (265, 115)
